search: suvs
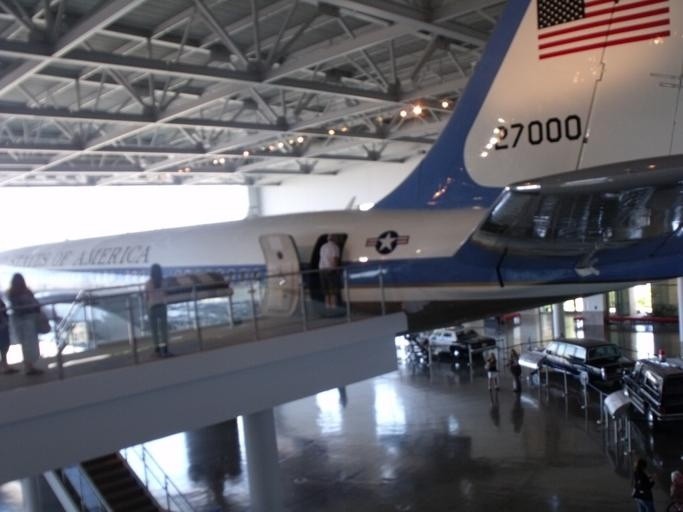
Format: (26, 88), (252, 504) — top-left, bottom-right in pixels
(425, 326), (683, 431)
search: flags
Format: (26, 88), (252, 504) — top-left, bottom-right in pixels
(536, 0), (670, 58)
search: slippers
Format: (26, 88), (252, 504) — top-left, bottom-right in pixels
(1, 368), (20, 374)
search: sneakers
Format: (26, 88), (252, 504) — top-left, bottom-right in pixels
(25, 369), (44, 375)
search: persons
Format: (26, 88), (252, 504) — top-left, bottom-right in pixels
(665, 470), (683, 512)
(8, 273), (45, 375)
(318, 234), (340, 308)
(145, 264), (175, 357)
(484, 353), (499, 391)
(512, 393), (525, 434)
(489, 391), (500, 426)
(505, 350), (522, 392)
(0, 298), (19, 375)
(631, 458), (657, 512)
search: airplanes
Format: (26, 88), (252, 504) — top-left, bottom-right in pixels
(1, 0), (683, 334)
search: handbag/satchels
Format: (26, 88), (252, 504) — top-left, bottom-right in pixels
(35, 313), (51, 333)
(484, 362), (490, 370)
(513, 366), (521, 376)
(631, 487), (644, 499)
